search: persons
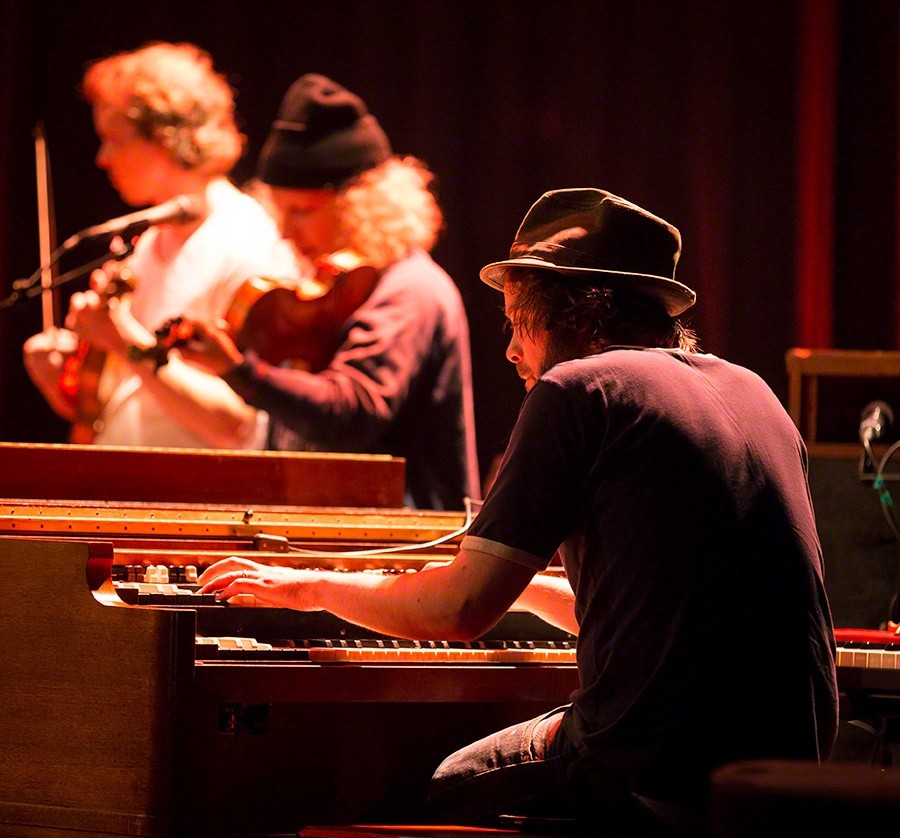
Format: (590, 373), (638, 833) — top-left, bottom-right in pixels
(198, 188), (841, 837)
(178, 73), (481, 514)
(24, 42), (324, 452)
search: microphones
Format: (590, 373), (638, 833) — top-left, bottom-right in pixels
(82, 193), (203, 239)
(860, 402), (894, 440)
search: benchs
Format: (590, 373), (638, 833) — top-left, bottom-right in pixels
(296, 813), (575, 837)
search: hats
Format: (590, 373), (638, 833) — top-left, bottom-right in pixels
(480, 187), (696, 317)
(257, 73), (391, 188)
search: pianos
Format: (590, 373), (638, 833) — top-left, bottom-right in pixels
(151, 605), (585, 838)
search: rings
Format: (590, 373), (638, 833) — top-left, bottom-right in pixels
(242, 568), (248, 577)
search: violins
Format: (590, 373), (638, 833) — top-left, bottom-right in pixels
(130, 250), (377, 376)
